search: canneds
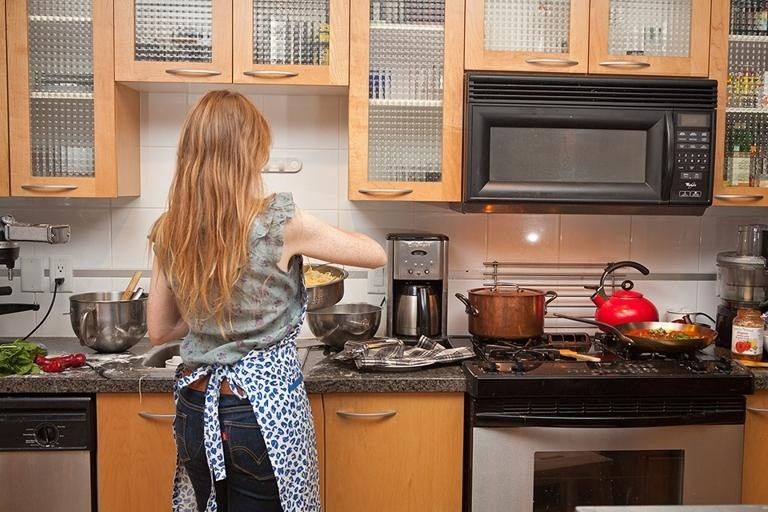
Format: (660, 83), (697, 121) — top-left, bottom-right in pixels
(732, 302), (765, 369)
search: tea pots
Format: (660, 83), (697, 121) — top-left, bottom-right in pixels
(587, 260), (661, 331)
(394, 282), (441, 338)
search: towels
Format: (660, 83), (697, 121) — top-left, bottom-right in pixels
(336, 334), (477, 369)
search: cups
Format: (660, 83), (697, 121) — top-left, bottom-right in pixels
(667, 309), (717, 325)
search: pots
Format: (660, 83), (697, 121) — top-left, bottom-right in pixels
(551, 312), (720, 351)
(455, 281), (558, 338)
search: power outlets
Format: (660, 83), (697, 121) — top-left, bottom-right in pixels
(49, 255), (72, 293)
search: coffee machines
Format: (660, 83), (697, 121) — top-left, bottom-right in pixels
(385, 230), (450, 346)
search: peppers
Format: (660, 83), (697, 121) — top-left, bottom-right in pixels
(34, 355), (97, 373)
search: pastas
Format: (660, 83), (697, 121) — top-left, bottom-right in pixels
(304, 270), (339, 289)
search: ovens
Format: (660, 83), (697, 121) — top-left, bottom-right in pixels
(463, 416), (746, 512)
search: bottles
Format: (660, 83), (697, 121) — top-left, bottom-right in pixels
(729, 307), (763, 361)
(728, 121), (758, 185)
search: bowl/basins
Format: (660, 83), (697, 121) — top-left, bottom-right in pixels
(67, 290), (151, 349)
(310, 302), (382, 348)
(298, 262), (349, 310)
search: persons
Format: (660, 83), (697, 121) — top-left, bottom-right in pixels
(147, 90), (387, 512)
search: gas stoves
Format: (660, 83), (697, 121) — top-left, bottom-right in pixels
(446, 333), (757, 396)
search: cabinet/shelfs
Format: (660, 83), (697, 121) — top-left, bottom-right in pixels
(741, 391), (768, 504)
(113, 0), (348, 95)
(348, 1), (463, 204)
(322, 393), (464, 512)
(466, 0), (711, 76)
(95, 388), (326, 512)
(711, 0), (768, 207)
(1, 0), (139, 199)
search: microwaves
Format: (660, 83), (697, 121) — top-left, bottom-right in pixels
(450, 72), (716, 217)
